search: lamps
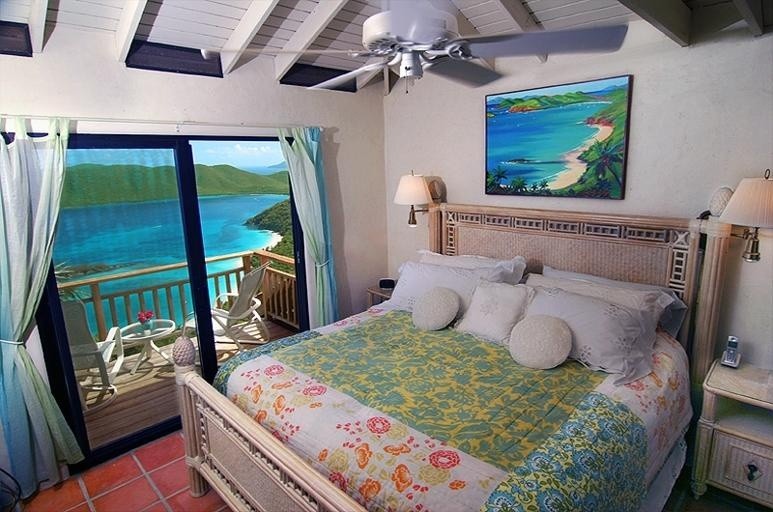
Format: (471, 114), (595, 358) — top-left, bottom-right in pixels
(718, 178), (773, 262)
(394, 175), (434, 226)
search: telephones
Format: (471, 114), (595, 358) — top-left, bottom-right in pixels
(719, 335), (742, 369)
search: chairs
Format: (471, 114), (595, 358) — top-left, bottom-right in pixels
(184, 262), (270, 367)
(62, 299), (124, 415)
(173, 299), (692, 511)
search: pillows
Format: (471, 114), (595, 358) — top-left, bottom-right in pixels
(522, 273), (674, 386)
(542, 264), (688, 338)
(390, 259), (514, 323)
(401, 249), (526, 286)
(510, 315), (574, 369)
(523, 286), (647, 376)
(412, 288), (458, 331)
(460, 279), (535, 345)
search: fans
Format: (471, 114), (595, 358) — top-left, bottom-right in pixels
(202, 4), (628, 111)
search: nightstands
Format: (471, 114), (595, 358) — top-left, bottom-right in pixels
(367, 282), (392, 310)
(690, 358), (772, 507)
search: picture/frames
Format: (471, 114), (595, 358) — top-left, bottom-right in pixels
(485, 74), (634, 199)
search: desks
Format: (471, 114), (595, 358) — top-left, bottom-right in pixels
(120, 319), (175, 375)
